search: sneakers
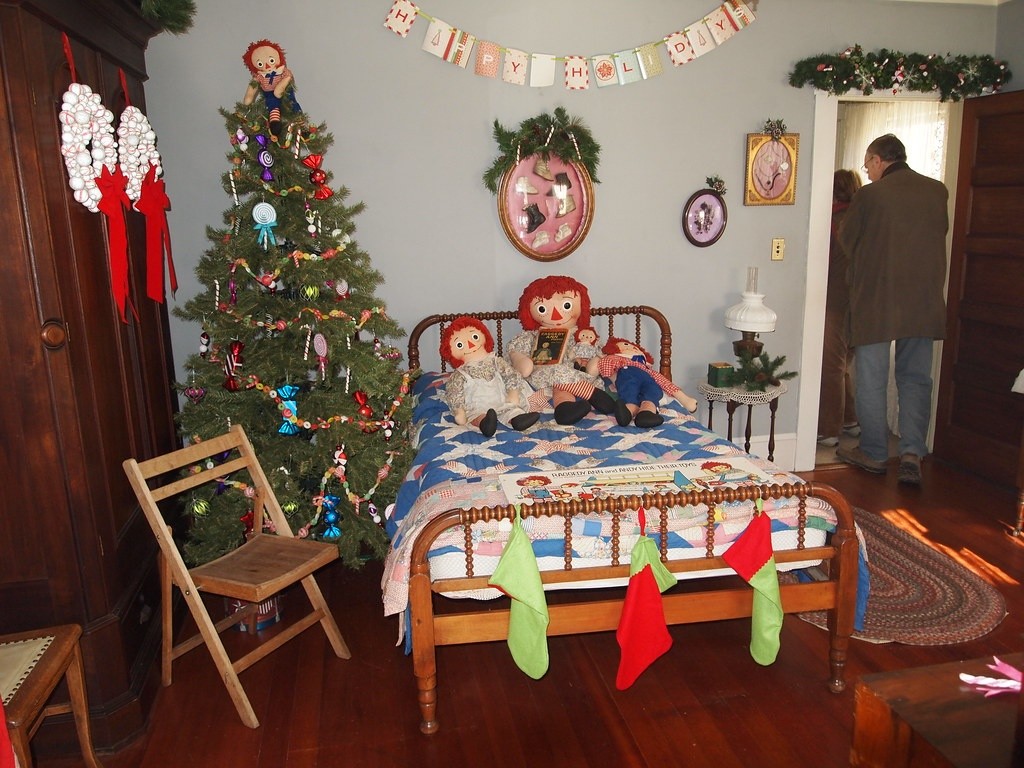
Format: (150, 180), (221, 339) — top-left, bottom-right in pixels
(897, 454), (923, 484)
(836, 446), (889, 474)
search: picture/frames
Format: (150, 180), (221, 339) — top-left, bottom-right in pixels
(496, 149), (595, 263)
(744, 133), (800, 206)
(530, 326), (571, 367)
(682, 190), (728, 248)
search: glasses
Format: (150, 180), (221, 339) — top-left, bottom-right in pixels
(860, 157), (873, 174)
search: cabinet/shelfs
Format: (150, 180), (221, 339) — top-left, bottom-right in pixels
(0, 0), (184, 758)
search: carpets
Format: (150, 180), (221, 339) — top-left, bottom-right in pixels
(776, 506), (1008, 648)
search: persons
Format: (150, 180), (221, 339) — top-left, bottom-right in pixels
(817, 169), (863, 446)
(835, 134), (950, 488)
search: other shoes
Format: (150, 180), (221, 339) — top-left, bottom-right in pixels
(817, 433), (839, 446)
(842, 422), (861, 437)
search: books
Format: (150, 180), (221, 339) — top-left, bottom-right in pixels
(530, 327), (570, 364)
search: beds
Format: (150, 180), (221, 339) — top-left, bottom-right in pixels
(385, 305), (859, 738)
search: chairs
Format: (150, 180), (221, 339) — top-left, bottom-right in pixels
(0, 624), (103, 768)
(121, 424), (352, 729)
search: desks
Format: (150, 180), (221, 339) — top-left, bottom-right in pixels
(859, 650), (1024, 768)
(697, 373), (787, 463)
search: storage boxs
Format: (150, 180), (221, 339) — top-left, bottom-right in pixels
(707, 362), (734, 388)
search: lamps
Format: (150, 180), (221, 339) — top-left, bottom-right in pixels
(725, 267), (776, 379)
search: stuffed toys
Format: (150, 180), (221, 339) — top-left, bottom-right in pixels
(505, 275), (698, 428)
(440, 318), (540, 438)
(242, 39), (301, 136)
